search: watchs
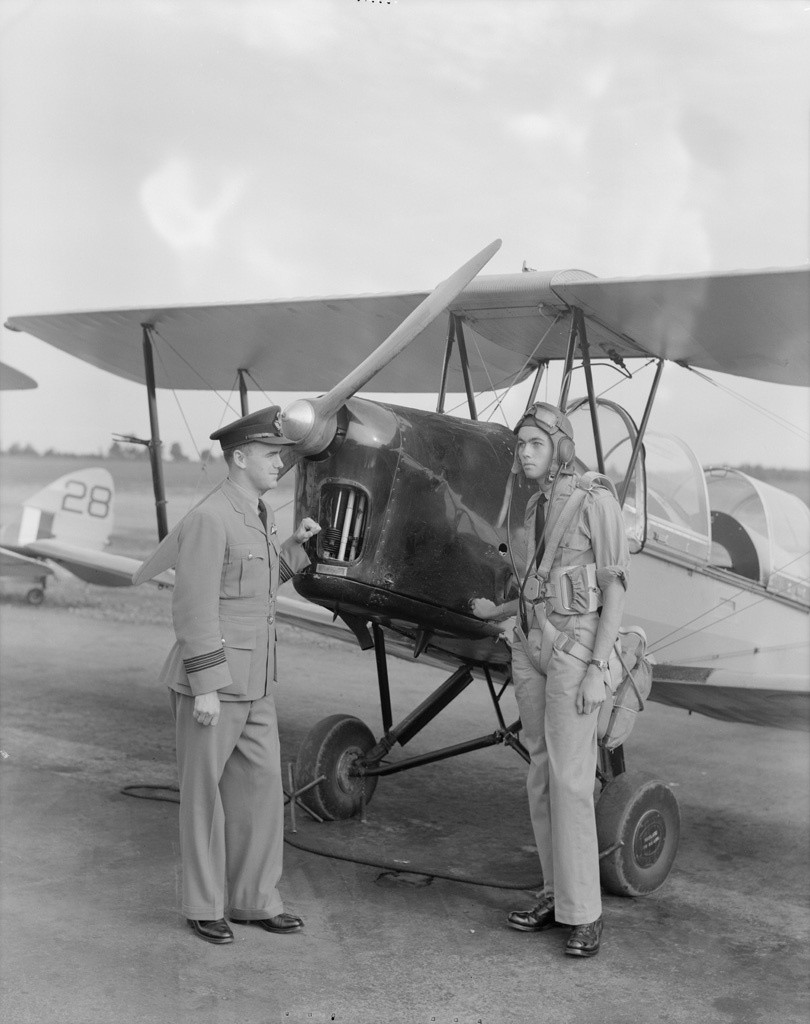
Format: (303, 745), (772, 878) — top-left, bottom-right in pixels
(591, 660), (608, 671)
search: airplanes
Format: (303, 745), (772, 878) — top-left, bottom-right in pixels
(1, 236), (809, 899)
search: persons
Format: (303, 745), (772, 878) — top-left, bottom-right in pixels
(469, 402), (628, 953)
(161, 405), (321, 945)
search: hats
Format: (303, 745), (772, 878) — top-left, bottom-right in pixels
(209, 406), (298, 450)
(512, 401), (575, 481)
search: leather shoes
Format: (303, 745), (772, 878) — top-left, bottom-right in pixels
(187, 918), (234, 943)
(506, 896), (560, 931)
(230, 912), (305, 932)
(565, 915), (603, 957)
